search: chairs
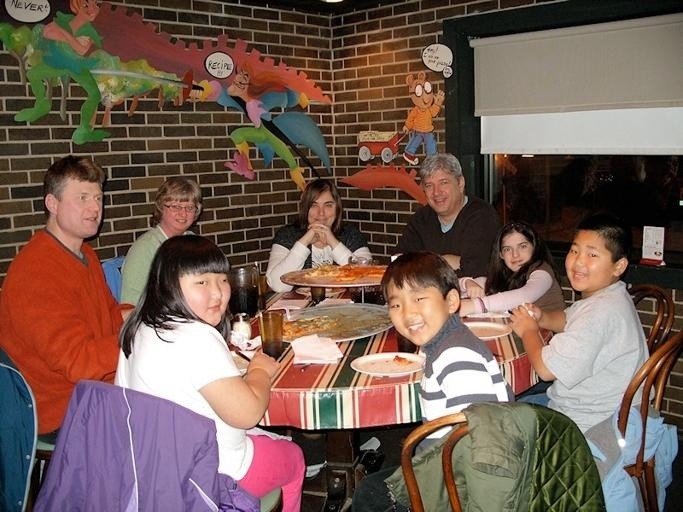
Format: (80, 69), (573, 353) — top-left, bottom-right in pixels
(399, 401), (608, 512)
(0, 349), (61, 512)
(29, 377), (285, 511)
(614, 327), (681, 512)
(627, 282), (675, 361)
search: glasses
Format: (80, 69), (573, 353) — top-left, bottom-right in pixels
(164, 204), (195, 211)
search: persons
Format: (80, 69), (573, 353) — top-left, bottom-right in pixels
(0, 156), (124, 445)
(352, 249), (516, 511)
(453, 221), (564, 322)
(263, 176), (371, 290)
(117, 174), (203, 322)
(505, 217), (655, 440)
(387, 151), (491, 280)
(108, 234), (306, 512)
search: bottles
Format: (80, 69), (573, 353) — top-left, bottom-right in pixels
(232, 313), (251, 341)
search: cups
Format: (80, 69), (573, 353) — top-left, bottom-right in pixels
(227, 267), (259, 320)
(257, 310), (284, 361)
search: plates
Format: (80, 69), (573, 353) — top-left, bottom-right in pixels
(461, 320), (513, 342)
(349, 353), (428, 378)
